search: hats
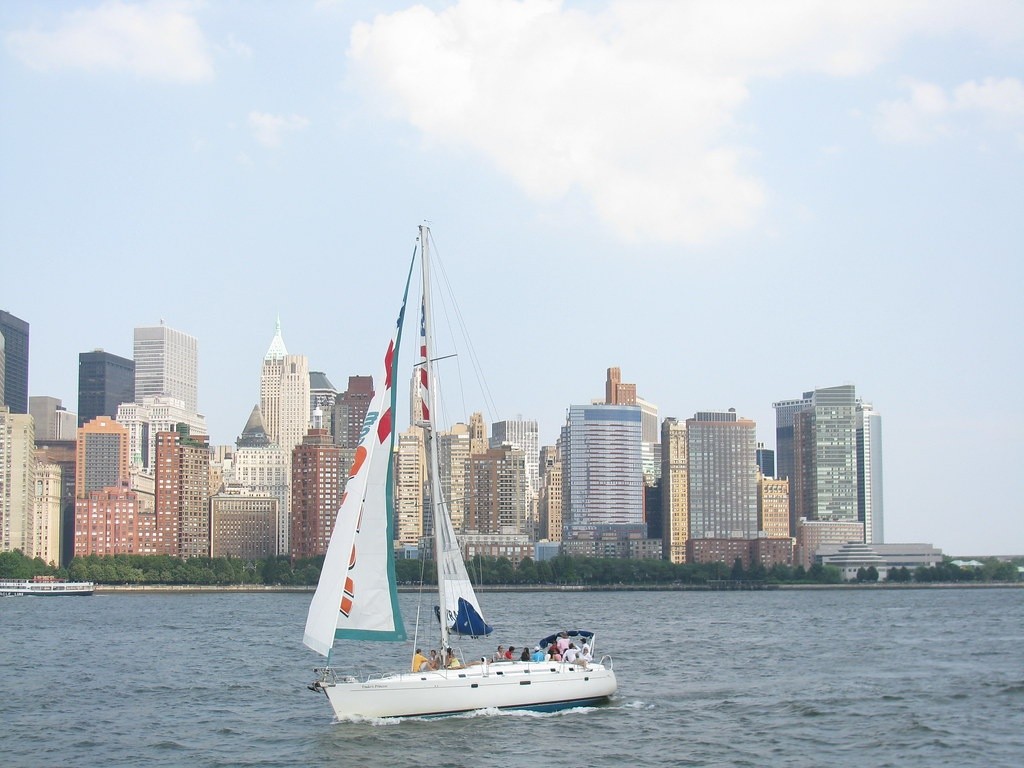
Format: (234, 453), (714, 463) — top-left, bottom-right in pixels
(535, 646), (540, 651)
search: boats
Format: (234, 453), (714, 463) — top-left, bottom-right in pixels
(0, 582), (95, 597)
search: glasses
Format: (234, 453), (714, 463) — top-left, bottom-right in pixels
(552, 642), (556, 644)
(500, 648), (503, 649)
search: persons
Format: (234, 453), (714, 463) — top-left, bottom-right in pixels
(414, 649), (433, 672)
(430, 650), (440, 670)
(505, 646), (514, 658)
(548, 632), (592, 665)
(462, 659), (492, 666)
(447, 654), (463, 670)
(496, 645), (504, 659)
(532, 647), (544, 661)
(521, 648), (530, 661)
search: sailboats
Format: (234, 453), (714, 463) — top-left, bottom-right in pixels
(299, 222), (620, 727)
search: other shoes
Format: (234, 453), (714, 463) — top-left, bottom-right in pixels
(588, 668), (593, 672)
(583, 669), (588, 672)
